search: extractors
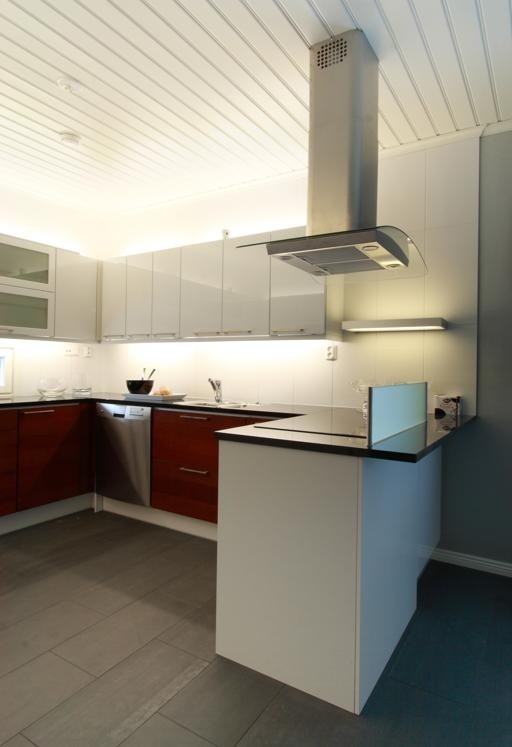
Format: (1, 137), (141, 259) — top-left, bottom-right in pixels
(236, 30), (427, 289)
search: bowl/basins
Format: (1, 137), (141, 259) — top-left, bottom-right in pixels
(37, 382), (93, 398)
(126, 379), (153, 394)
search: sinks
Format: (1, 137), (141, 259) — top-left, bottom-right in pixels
(175, 400), (249, 409)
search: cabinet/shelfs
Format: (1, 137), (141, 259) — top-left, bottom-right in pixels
(102, 225), (327, 340)
(152, 409), (283, 542)
(0, 235), (54, 339)
(1, 405), (91, 538)
(55, 251), (101, 342)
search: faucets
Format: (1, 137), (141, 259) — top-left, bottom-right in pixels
(208, 378), (223, 404)
